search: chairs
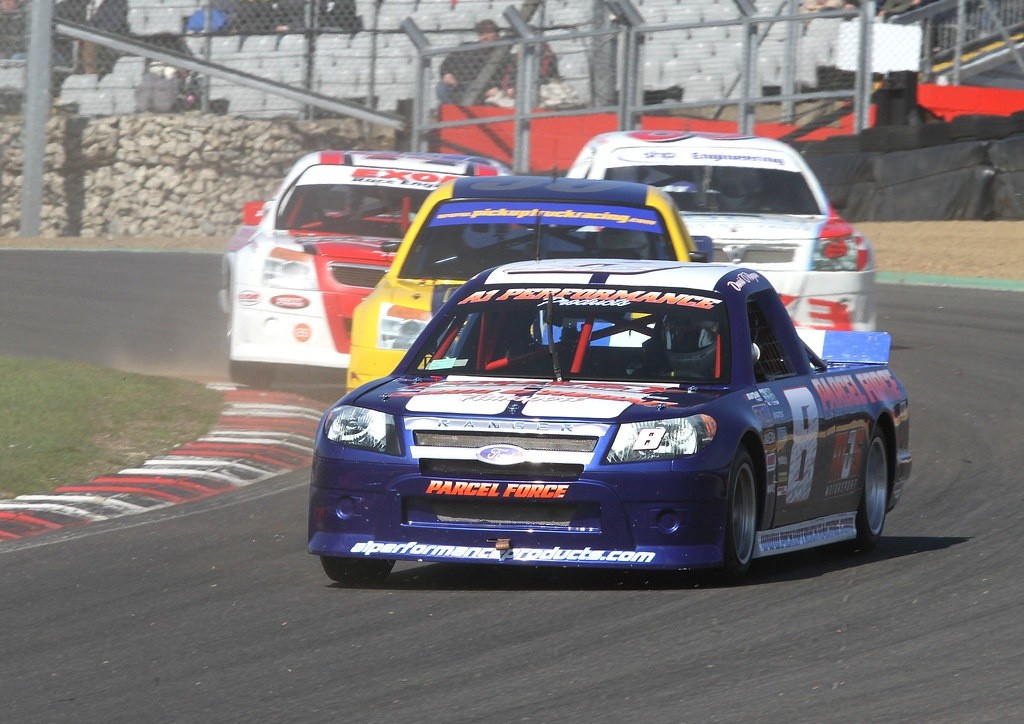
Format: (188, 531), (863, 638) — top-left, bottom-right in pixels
(59, 0), (843, 114)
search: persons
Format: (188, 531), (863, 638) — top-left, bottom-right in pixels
(437, 19), (567, 107)
(183, 0), (364, 36)
(660, 313), (719, 379)
(595, 227), (651, 260)
(0, 1), (227, 115)
(796, 0), (1024, 47)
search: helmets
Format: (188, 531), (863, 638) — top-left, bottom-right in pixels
(596, 227), (651, 261)
(665, 312), (719, 370)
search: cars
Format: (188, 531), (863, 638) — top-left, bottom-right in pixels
(220, 149), (518, 392)
(343, 175), (713, 400)
(306, 257), (914, 587)
(563, 127), (878, 335)
